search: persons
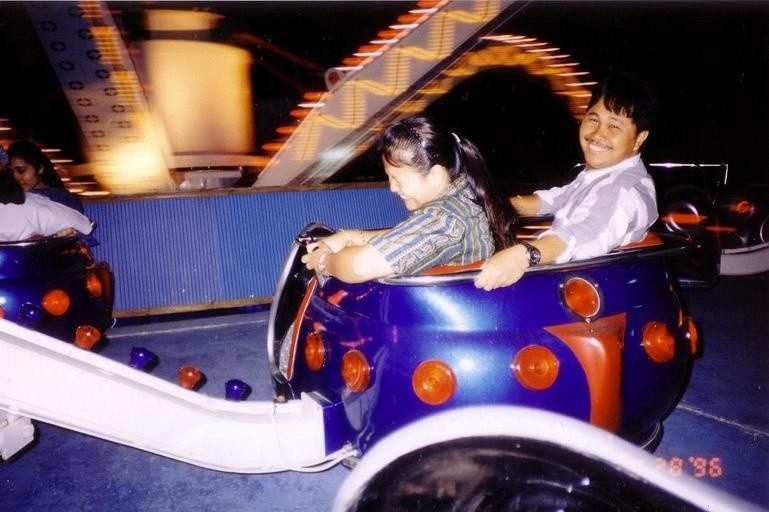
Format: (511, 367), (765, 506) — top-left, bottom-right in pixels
(299, 117), (519, 286)
(5, 139), (86, 216)
(0, 142), (97, 243)
(473, 73), (660, 293)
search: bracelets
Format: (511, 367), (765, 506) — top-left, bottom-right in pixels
(352, 228), (367, 247)
(317, 251), (335, 277)
(516, 193), (528, 217)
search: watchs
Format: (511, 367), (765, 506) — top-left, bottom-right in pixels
(520, 240), (543, 268)
